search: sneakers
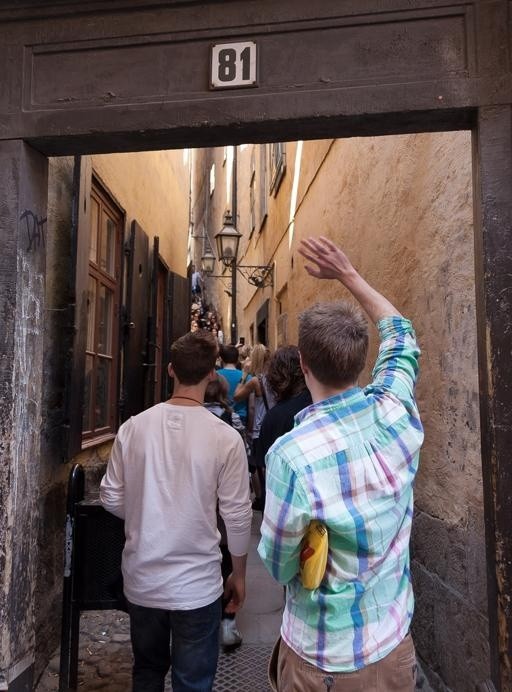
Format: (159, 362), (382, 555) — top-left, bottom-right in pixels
(221, 618), (242, 646)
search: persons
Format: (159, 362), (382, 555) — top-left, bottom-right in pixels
(99, 330), (249, 690)
(205, 373), (250, 647)
(189, 270), (252, 369)
(255, 345), (311, 499)
(233, 343), (277, 511)
(216, 346), (256, 432)
(257, 237), (425, 692)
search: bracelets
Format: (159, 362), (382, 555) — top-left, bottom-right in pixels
(239, 378), (246, 384)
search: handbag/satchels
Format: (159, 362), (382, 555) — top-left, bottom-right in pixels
(299, 520), (329, 590)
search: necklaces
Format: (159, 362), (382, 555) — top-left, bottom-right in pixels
(171, 395), (202, 406)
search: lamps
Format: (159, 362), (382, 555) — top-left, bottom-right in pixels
(216, 210), (277, 292)
(200, 244), (236, 298)
